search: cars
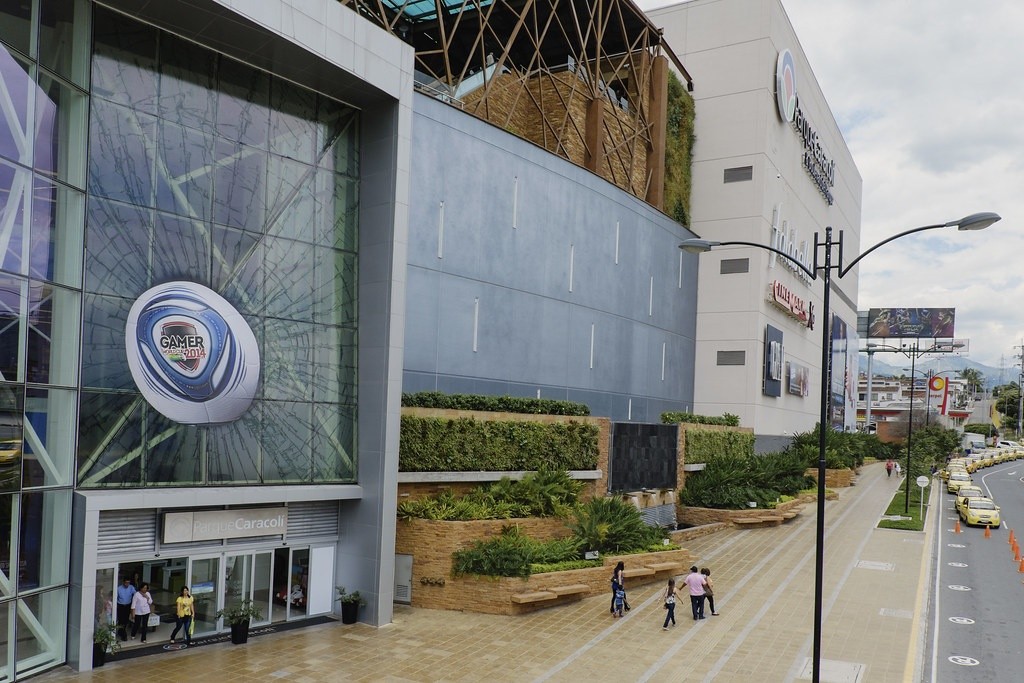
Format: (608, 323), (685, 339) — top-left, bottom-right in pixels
(996, 439), (1019, 448)
(940, 449), (1024, 528)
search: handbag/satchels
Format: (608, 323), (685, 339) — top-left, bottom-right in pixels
(664, 603), (667, 609)
(189, 617), (195, 635)
(705, 589), (712, 596)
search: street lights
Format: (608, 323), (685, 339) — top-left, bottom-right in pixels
(676, 212), (1001, 683)
(865, 342), (966, 513)
(902, 368), (962, 433)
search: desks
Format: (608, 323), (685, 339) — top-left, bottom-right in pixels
(143, 560), (168, 584)
(162, 565), (186, 590)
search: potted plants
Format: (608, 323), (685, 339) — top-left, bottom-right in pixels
(215, 598), (264, 644)
(93, 620), (123, 668)
(334, 585), (366, 625)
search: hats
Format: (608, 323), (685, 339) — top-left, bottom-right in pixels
(689, 566), (697, 571)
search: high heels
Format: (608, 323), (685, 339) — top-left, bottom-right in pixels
(611, 608), (615, 613)
(624, 606), (630, 611)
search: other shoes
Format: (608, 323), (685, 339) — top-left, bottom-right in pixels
(613, 613), (617, 618)
(663, 627), (668, 631)
(619, 615), (623, 617)
(700, 616), (706, 619)
(712, 613), (719, 615)
(142, 640), (146, 643)
(170, 640), (175, 643)
(131, 637), (136, 639)
(673, 624), (676, 627)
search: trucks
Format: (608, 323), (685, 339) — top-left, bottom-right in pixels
(960, 431), (986, 454)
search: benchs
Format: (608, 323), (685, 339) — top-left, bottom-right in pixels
(733, 505), (806, 525)
(512, 593), (557, 605)
(645, 562), (681, 571)
(547, 584), (591, 595)
(623, 568), (653, 578)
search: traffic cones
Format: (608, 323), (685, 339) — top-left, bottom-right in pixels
(955, 520), (961, 533)
(985, 525), (992, 538)
(1018, 556), (1024, 574)
(1008, 530), (1021, 560)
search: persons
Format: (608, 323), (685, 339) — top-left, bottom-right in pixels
(610, 561), (719, 630)
(885, 458), (902, 477)
(96, 572), (196, 645)
(930, 465), (942, 479)
(278, 567), (308, 607)
